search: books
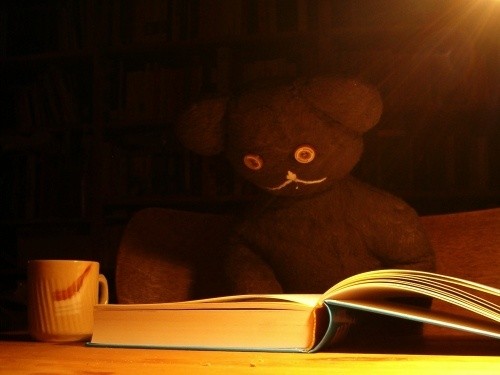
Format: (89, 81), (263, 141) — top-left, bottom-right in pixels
(85, 269), (500, 354)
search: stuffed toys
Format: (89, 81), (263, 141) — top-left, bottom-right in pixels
(174, 71), (437, 309)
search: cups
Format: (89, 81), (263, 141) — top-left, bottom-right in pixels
(26, 259), (109, 344)
